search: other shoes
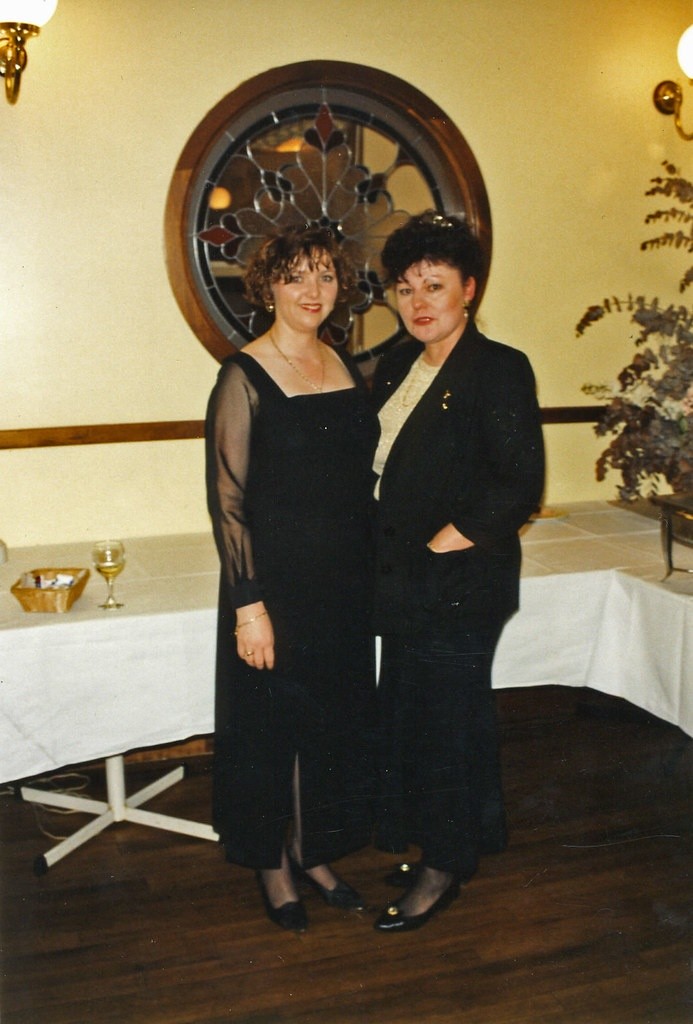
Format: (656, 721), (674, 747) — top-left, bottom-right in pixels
(376, 863), (483, 933)
(255, 865), (364, 935)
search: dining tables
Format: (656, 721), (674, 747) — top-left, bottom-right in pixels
(0, 492), (693, 872)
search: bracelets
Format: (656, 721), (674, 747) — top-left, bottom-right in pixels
(233, 611), (268, 636)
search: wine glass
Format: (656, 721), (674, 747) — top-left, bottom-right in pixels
(92, 540), (127, 609)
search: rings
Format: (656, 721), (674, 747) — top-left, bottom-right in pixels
(245, 651), (252, 658)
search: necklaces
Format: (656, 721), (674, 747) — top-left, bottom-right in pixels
(401, 352), (423, 407)
(268, 329), (326, 394)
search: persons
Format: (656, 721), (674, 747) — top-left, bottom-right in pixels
(204, 225), (382, 932)
(361, 206), (549, 937)
